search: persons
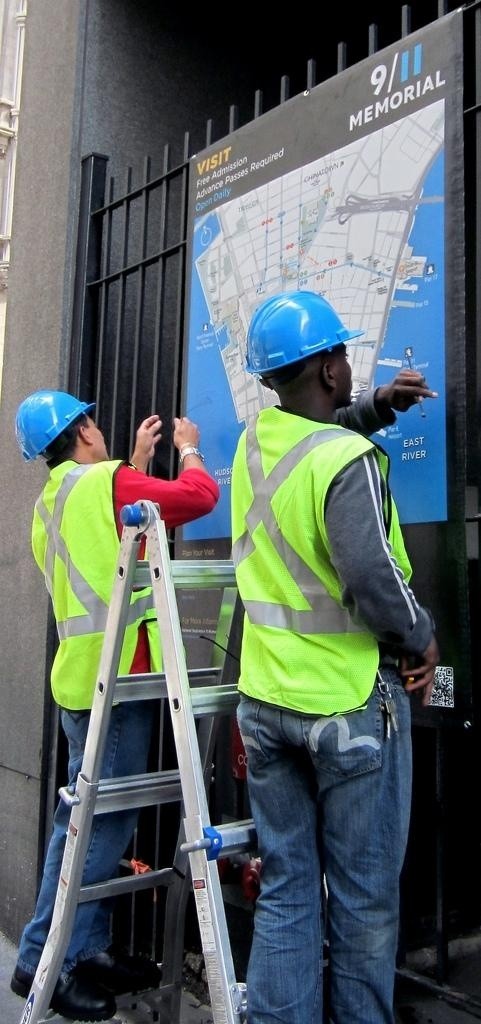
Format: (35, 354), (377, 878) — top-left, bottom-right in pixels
(223, 288), (443, 1024)
(12, 388), (221, 1024)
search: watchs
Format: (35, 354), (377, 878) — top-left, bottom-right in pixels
(178, 446), (204, 464)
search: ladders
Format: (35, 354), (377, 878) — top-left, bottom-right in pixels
(16, 501), (260, 1023)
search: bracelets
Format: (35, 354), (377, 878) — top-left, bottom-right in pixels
(179, 441), (198, 453)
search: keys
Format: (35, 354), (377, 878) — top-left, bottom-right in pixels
(376, 700), (396, 743)
(384, 695), (400, 735)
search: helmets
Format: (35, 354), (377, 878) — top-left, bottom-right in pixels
(16, 389), (94, 461)
(244, 291), (366, 376)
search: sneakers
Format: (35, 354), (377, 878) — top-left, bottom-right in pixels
(10, 963), (116, 1022)
(68, 946), (164, 993)
